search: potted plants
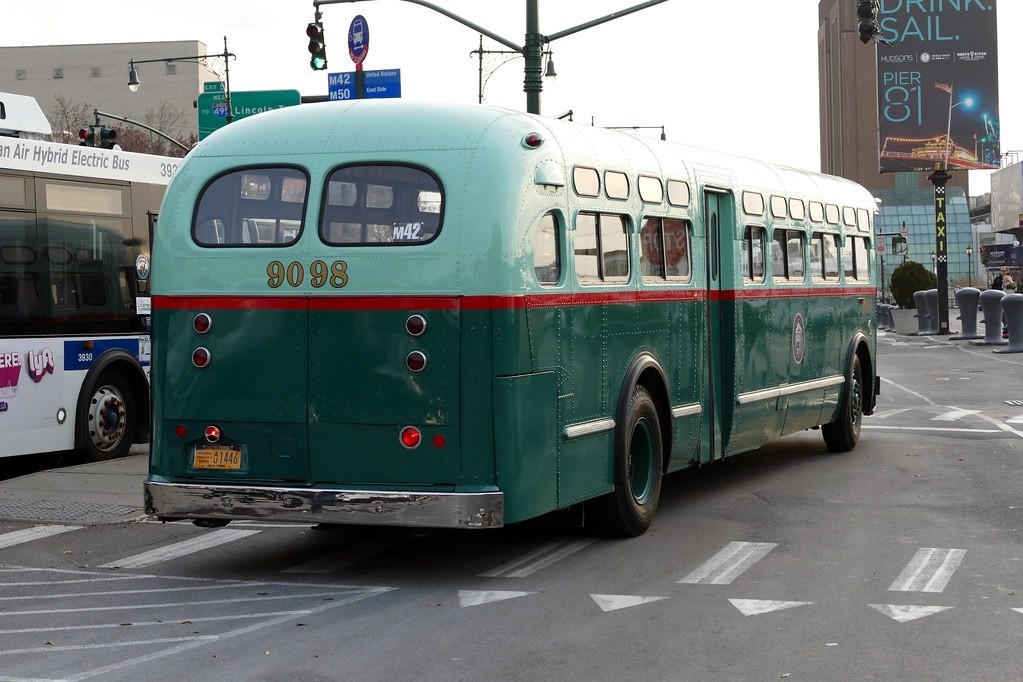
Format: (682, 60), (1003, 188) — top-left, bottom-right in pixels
(890, 260), (934, 335)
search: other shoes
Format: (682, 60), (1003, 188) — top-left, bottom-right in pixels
(1002, 332), (1008, 338)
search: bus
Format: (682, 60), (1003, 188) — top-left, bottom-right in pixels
(0, 135), (258, 459)
(144, 94), (909, 538)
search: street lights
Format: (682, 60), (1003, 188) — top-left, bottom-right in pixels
(966, 243), (973, 289)
(930, 251), (938, 273)
(125, 35), (237, 126)
(881, 260), (888, 298)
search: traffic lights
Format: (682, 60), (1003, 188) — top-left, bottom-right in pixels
(306, 21), (329, 73)
(79, 127), (94, 147)
(99, 127), (118, 150)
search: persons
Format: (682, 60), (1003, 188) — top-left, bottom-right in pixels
(991, 266), (1017, 340)
